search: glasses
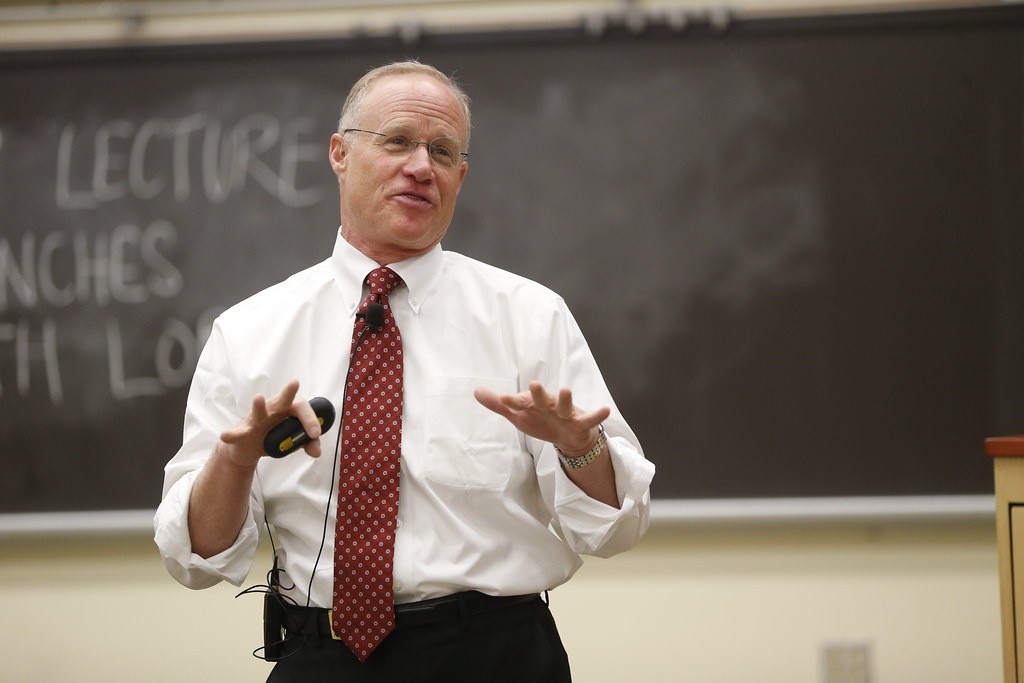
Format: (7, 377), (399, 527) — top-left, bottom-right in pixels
(344, 129), (470, 168)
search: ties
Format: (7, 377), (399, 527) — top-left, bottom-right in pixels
(333, 270), (402, 656)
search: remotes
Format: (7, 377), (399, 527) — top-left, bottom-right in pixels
(264, 398), (336, 459)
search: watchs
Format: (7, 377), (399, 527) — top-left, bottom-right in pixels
(554, 421), (607, 470)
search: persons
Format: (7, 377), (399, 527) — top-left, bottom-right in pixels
(153, 59), (656, 682)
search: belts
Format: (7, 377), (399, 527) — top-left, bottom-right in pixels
(282, 595), (530, 644)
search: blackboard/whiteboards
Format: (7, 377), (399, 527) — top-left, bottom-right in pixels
(0, 2), (1024, 517)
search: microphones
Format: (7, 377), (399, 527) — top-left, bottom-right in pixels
(365, 303), (385, 332)
